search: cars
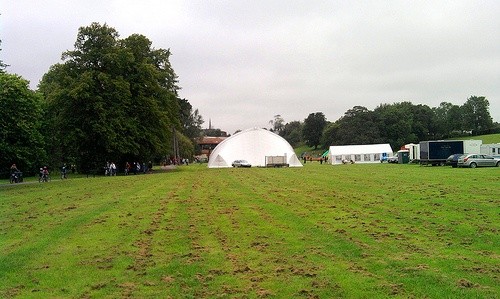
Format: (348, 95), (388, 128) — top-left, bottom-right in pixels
(446, 153), (500, 168)
(387, 153), (399, 163)
(232, 160), (251, 168)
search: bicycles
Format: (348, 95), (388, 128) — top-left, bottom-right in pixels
(10, 171), (23, 184)
(125, 166), (149, 175)
(39, 172), (49, 182)
(60, 166), (67, 179)
(103, 166), (116, 176)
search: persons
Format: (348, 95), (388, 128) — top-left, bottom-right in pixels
(302, 153), (329, 164)
(9, 163), (69, 182)
(105, 161), (153, 177)
(162, 156), (199, 167)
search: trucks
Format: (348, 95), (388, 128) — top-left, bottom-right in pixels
(405, 140), (500, 166)
